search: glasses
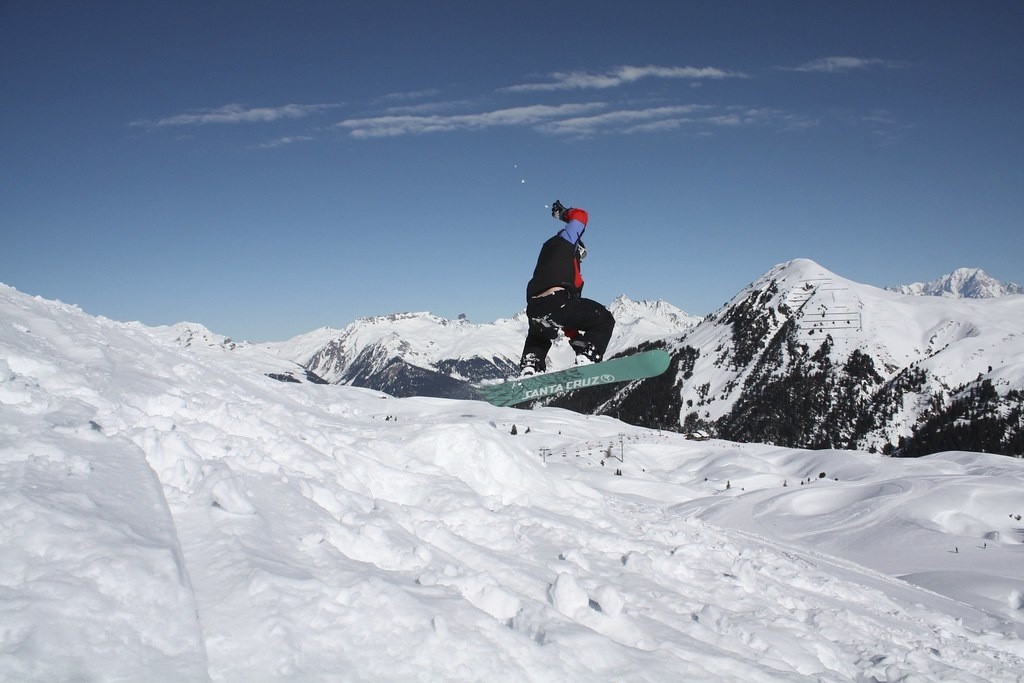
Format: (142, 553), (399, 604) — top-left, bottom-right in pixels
(577, 245), (587, 263)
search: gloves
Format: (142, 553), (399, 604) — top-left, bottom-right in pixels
(551, 200), (573, 221)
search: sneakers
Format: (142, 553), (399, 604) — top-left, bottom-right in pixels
(569, 334), (603, 363)
(519, 351), (546, 377)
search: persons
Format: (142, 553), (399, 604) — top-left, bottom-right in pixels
(518, 200), (615, 377)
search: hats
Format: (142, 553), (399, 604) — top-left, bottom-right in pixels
(578, 240), (585, 248)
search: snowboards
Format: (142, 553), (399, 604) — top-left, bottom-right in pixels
(473, 348), (670, 408)
